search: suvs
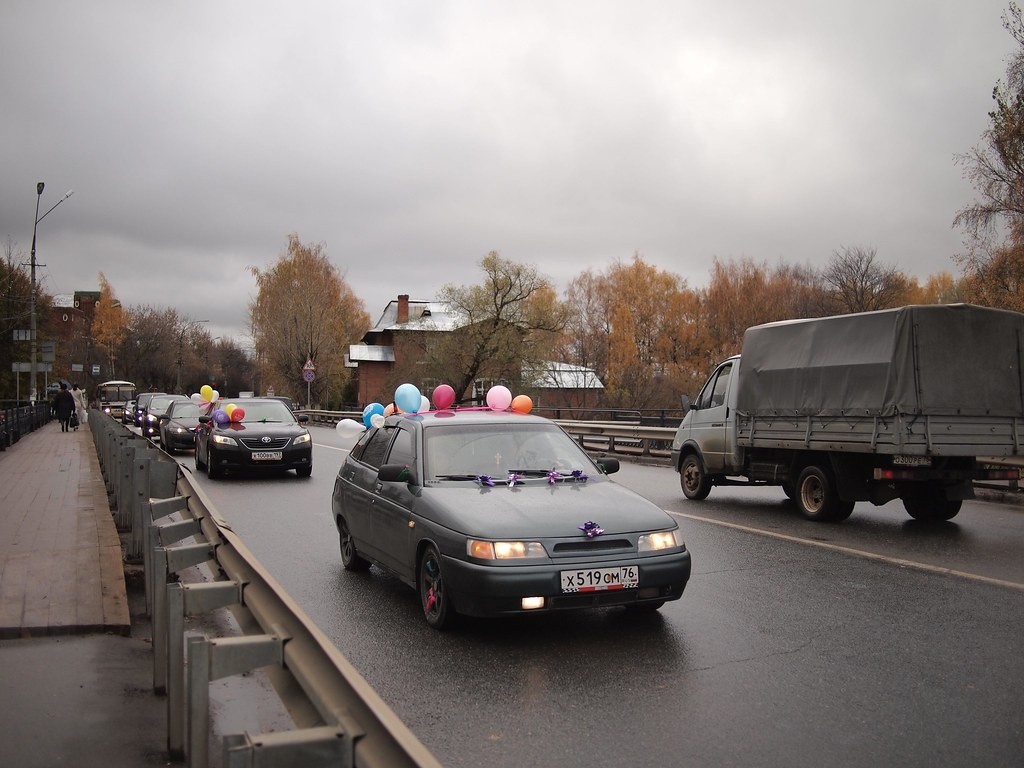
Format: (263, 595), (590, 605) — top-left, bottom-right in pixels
(138, 394), (191, 440)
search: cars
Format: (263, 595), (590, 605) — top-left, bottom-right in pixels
(122, 400), (137, 424)
(131, 393), (167, 427)
(194, 398), (313, 481)
(159, 400), (205, 456)
(331, 411), (691, 633)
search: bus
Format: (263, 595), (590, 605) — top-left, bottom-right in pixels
(96, 380), (136, 418)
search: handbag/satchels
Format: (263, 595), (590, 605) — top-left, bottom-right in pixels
(70, 413), (79, 427)
(81, 410), (88, 424)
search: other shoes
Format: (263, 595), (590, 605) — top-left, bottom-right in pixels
(76, 427), (78, 429)
(66, 429), (68, 432)
(74, 429), (75, 431)
(62, 425), (64, 432)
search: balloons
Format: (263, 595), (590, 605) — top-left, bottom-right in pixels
(511, 393), (533, 413)
(190, 385), (219, 415)
(231, 408), (245, 422)
(394, 383), (422, 414)
(335, 401), (398, 438)
(417, 394), (431, 413)
(213, 410), (229, 423)
(432, 384), (456, 410)
(486, 384), (513, 411)
(225, 404), (237, 420)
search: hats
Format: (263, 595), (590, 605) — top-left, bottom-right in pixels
(59, 384), (68, 389)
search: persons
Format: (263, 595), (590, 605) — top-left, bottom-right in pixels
(53, 383), (87, 432)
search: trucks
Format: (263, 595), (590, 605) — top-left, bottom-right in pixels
(670, 303), (1024, 524)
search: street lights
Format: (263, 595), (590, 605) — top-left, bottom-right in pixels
(177, 320), (210, 393)
(211, 337), (220, 347)
(31, 182), (73, 401)
